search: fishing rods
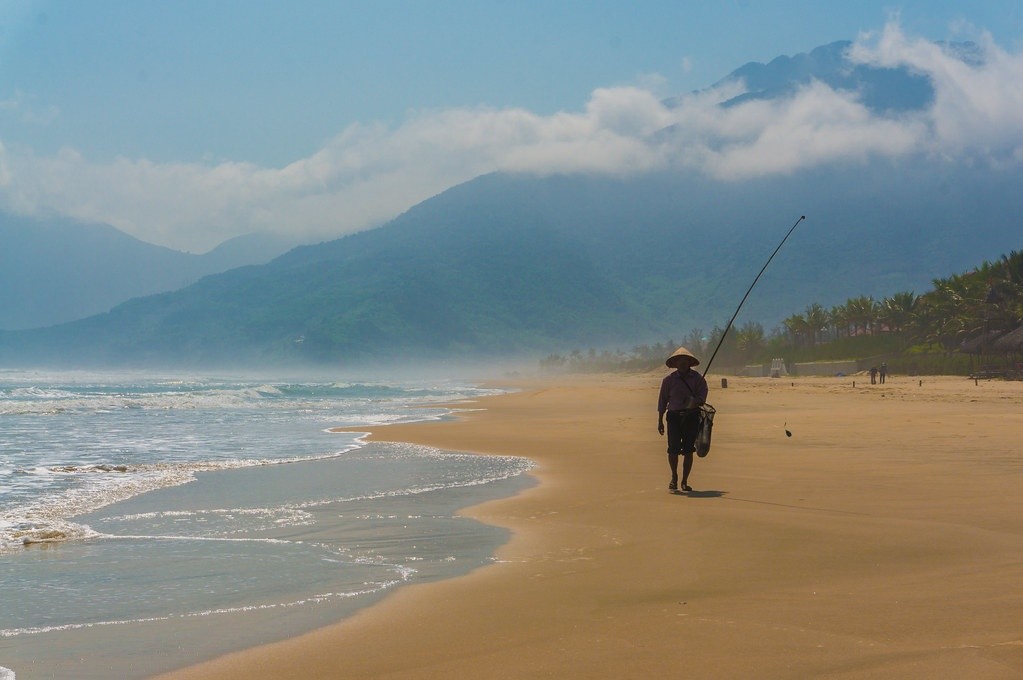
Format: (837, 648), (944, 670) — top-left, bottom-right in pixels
(679, 214), (806, 427)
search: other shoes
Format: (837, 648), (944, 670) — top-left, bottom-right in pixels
(668, 476), (678, 490)
(681, 481), (693, 492)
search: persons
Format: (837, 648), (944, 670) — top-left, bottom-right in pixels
(657, 347), (708, 491)
(867, 361), (889, 384)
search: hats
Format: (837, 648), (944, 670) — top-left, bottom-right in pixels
(665, 346), (701, 368)
